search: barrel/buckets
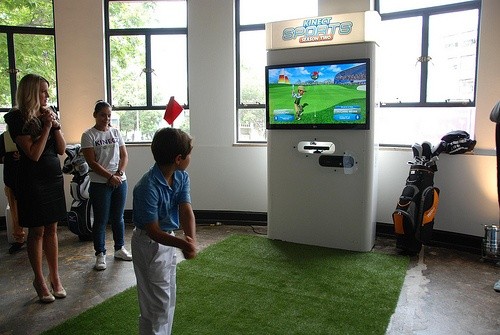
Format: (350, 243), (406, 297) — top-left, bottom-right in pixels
(484, 224), (500, 263)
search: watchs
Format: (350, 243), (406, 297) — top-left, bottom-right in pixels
(116, 170), (123, 176)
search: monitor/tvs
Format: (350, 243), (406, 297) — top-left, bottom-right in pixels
(265, 58), (370, 130)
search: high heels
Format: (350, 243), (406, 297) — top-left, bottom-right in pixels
(33, 274), (56, 302)
(46, 273), (66, 297)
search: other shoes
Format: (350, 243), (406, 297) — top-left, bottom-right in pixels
(8, 242), (25, 254)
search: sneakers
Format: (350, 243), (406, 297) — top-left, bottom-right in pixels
(114, 246), (132, 260)
(95, 252), (106, 270)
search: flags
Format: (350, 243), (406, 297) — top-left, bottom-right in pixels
(164, 97), (183, 124)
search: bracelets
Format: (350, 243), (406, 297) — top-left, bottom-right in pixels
(108, 174), (113, 180)
(52, 123), (60, 131)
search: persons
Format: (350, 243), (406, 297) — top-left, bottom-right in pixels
(0, 113), (23, 254)
(489, 100), (500, 291)
(8, 74), (67, 302)
(131, 127), (197, 335)
(81, 100), (133, 270)
